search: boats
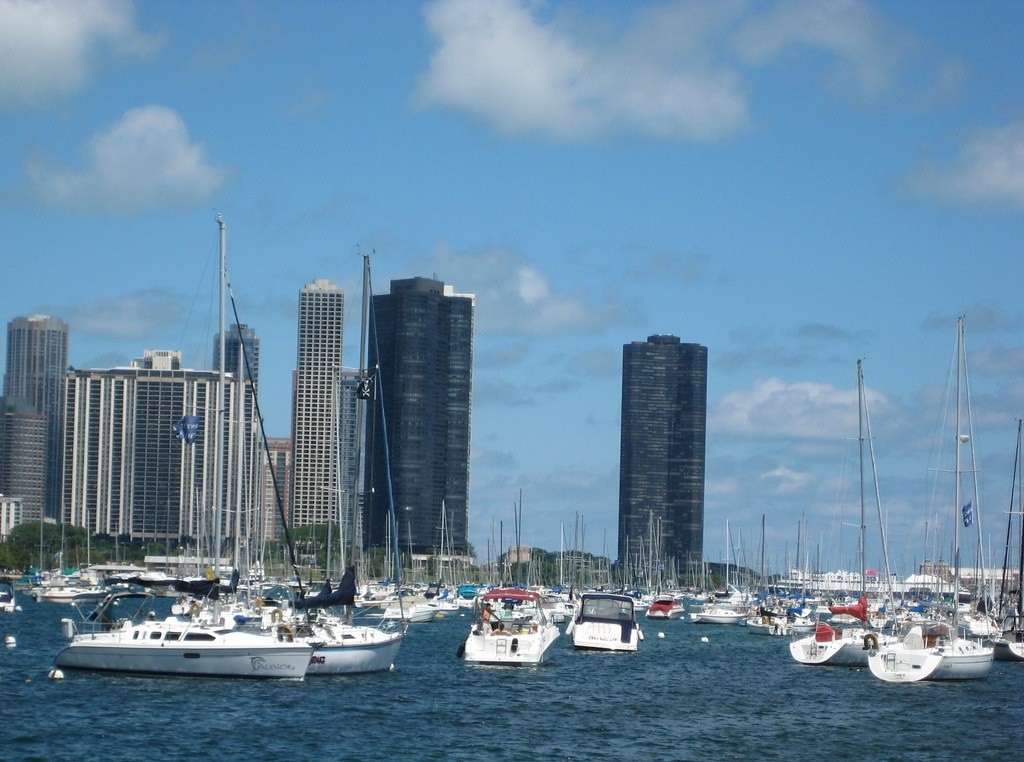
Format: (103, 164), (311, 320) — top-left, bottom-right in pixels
(565, 594), (645, 652)
(0, 577), (15, 613)
(457, 589), (560, 667)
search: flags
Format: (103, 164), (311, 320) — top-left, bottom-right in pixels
(357, 374), (377, 400)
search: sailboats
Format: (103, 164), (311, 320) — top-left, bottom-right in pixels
(747, 313), (1024, 682)
(457, 487), (747, 624)
(17, 212), (459, 681)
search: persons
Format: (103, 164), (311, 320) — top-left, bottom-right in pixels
(531, 622), (538, 633)
(492, 622), (510, 635)
(478, 602), (491, 635)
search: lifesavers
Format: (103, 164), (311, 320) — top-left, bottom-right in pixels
(253, 598), (262, 608)
(189, 604), (200, 618)
(865, 633), (878, 650)
(277, 625), (294, 643)
(271, 609), (283, 623)
